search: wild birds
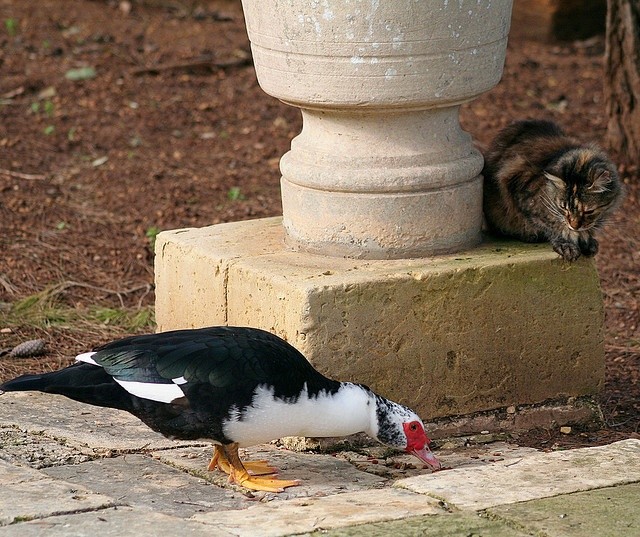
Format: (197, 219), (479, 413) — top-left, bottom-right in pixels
(0, 327), (442, 493)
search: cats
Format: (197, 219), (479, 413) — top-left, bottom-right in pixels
(483, 118), (626, 266)
